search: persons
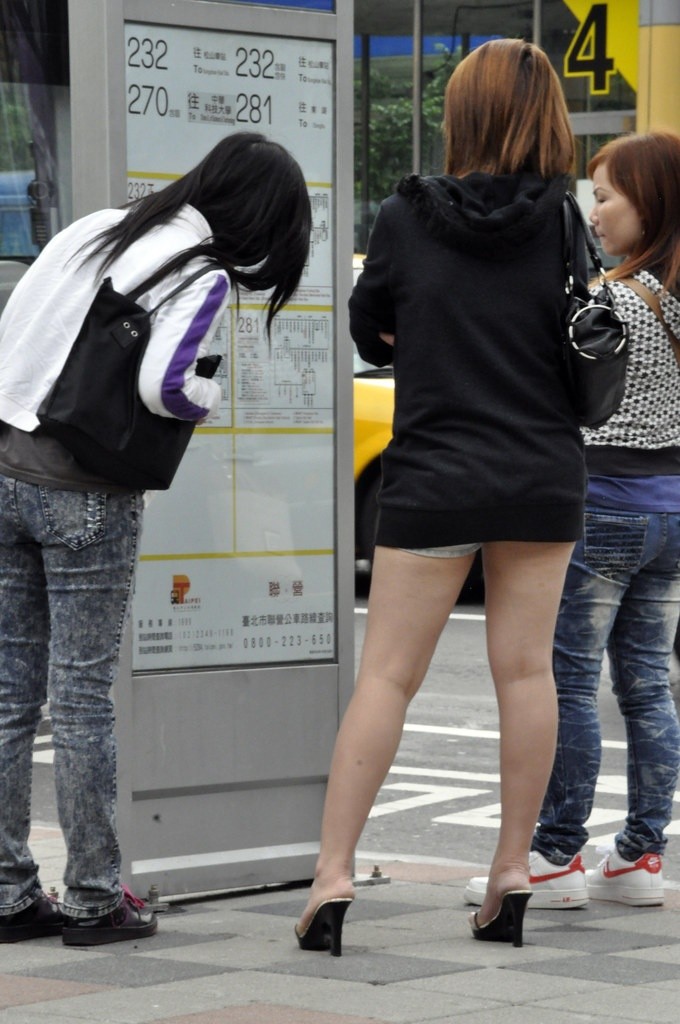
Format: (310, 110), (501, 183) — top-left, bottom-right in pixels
(466, 133), (679, 910)
(0, 134), (313, 947)
(293, 38), (588, 958)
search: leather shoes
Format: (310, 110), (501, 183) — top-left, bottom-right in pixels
(59, 884), (158, 947)
(0, 892), (65, 942)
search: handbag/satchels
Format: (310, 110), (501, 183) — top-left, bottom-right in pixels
(554, 188), (628, 432)
(41, 261), (241, 492)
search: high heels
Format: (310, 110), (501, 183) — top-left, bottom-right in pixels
(469, 889), (532, 947)
(295, 894), (352, 959)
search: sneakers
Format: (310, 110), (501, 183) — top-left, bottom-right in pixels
(583, 844), (665, 908)
(465, 851), (589, 910)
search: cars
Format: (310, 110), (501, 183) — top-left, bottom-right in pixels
(354, 251), (487, 608)
(0, 170), (56, 310)
